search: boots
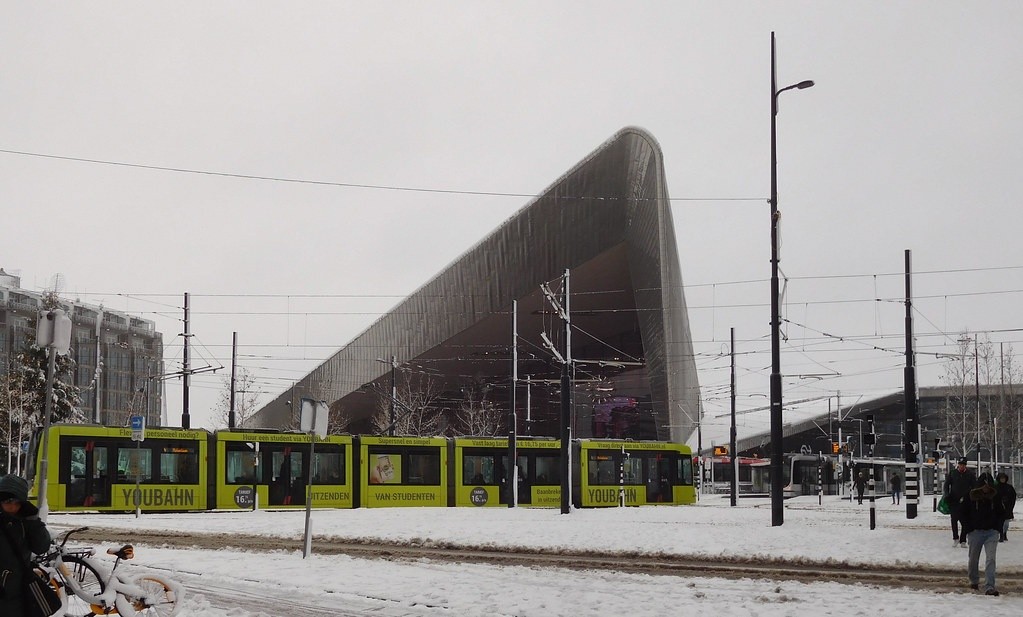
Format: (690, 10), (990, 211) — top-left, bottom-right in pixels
(999, 532), (1004, 542)
(1004, 532), (1008, 541)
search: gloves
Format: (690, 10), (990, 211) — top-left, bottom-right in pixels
(944, 495), (952, 504)
(22, 501), (39, 518)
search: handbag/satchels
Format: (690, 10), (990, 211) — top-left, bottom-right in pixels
(22, 570), (61, 617)
(937, 495), (951, 514)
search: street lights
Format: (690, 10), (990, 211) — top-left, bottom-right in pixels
(767, 32), (816, 527)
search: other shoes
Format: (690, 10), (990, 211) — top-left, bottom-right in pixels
(970, 583), (979, 592)
(961, 542), (967, 548)
(985, 589), (1000, 597)
(952, 539), (960, 547)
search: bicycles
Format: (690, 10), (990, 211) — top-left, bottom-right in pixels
(32, 525), (183, 617)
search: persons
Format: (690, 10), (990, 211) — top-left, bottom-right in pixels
(853, 472), (867, 504)
(958, 473), (1005, 596)
(944, 458), (976, 547)
(995, 473), (1016, 542)
(0, 474), (51, 617)
(890, 473), (900, 505)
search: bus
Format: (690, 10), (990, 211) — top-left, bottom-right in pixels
(21, 424), (700, 514)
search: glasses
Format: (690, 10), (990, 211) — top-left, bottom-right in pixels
(0, 497), (19, 503)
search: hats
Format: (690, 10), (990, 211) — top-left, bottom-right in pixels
(0, 474), (28, 503)
(958, 457), (967, 465)
